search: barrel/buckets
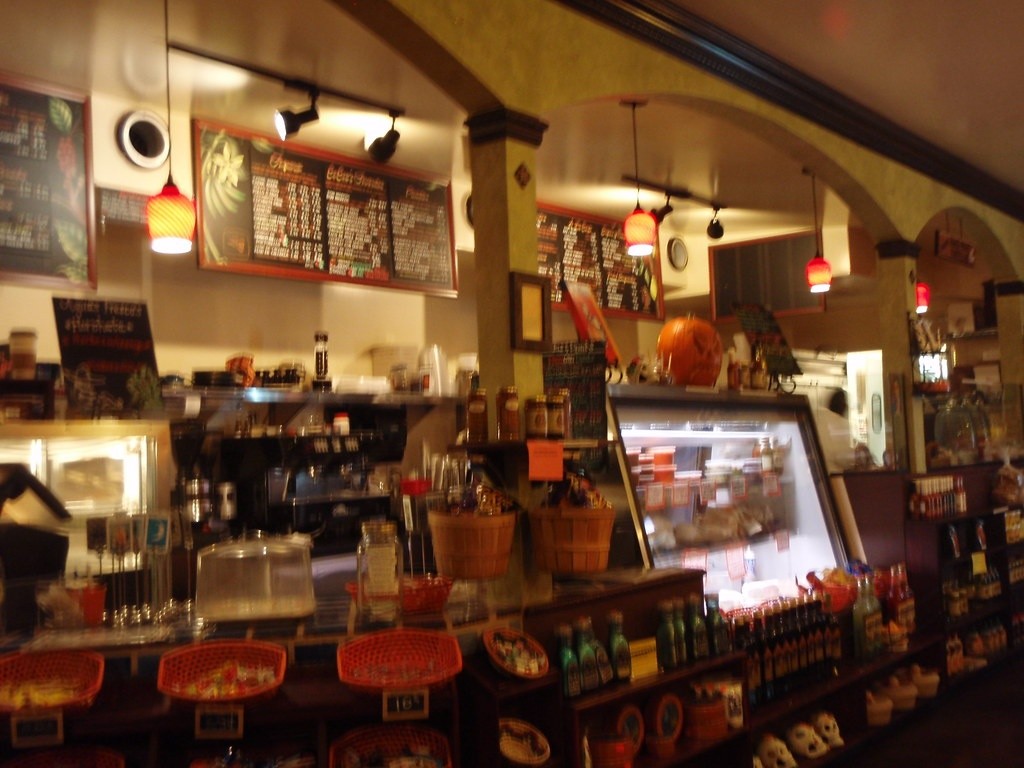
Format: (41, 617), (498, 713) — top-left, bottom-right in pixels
(427, 510), (515, 581)
(528, 506), (616, 574)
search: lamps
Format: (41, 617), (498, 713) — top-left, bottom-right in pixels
(620, 98), (656, 256)
(368, 112), (400, 163)
(802, 169), (832, 293)
(650, 191), (673, 223)
(146, 0), (196, 254)
(706, 204), (724, 238)
(914, 278), (931, 315)
(274, 89), (321, 141)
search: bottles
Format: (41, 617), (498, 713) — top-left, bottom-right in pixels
(253, 368), (300, 392)
(659, 558), (915, 710)
(727, 360), (766, 391)
(466, 388), (489, 443)
(770, 436), (784, 475)
(524, 387), (572, 441)
(356, 522), (401, 622)
(742, 545), (755, 582)
(639, 362), (673, 385)
(553, 609), (631, 701)
(907, 474), (967, 521)
(495, 385), (520, 441)
(334, 412), (350, 436)
(940, 558), (1024, 676)
(759, 437), (774, 474)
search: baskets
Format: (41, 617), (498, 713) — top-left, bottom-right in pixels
(157, 638), (286, 703)
(347, 574), (453, 616)
(483, 624), (550, 678)
(1, 648), (106, 712)
(499, 718), (550, 765)
(336, 626), (462, 693)
(330, 722), (452, 768)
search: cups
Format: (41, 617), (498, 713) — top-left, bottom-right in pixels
(9, 331), (38, 380)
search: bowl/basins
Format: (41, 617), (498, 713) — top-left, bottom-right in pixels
(624, 445), (762, 487)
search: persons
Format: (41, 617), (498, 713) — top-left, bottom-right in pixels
(935, 364), (991, 469)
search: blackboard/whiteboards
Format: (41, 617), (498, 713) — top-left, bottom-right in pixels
(0, 71), (99, 295)
(535, 198), (668, 323)
(190, 117), (461, 300)
(709, 229), (824, 316)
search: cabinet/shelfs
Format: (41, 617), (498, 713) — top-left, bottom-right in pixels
(0, 387), (1024, 768)
